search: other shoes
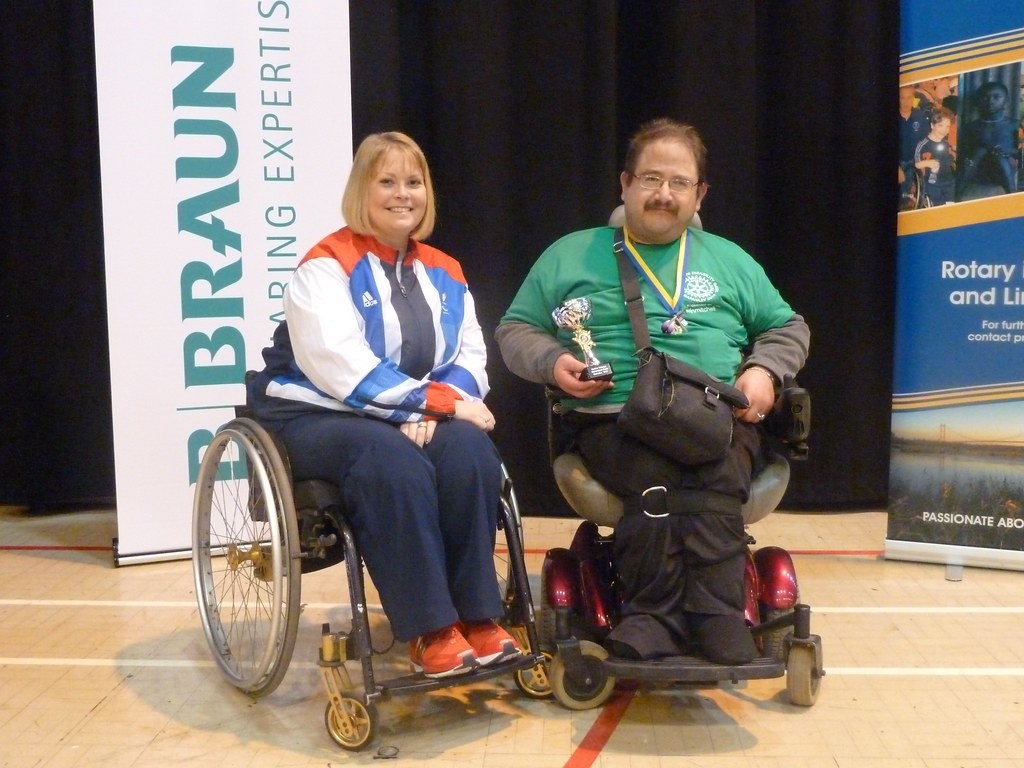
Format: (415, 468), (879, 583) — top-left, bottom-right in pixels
(612, 642), (640, 658)
(693, 613), (756, 664)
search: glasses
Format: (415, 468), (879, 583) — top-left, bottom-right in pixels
(632, 173), (700, 192)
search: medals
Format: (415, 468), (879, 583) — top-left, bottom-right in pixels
(660, 312), (689, 336)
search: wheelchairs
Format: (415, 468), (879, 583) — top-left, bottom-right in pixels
(534, 376), (830, 710)
(189, 370), (561, 751)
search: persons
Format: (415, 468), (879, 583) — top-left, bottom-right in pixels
(898, 72), (1024, 207)
(496, 116), (810, 664)
(250, 130), (523, 679)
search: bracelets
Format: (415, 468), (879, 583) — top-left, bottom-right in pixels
(745, 365), (777, 387)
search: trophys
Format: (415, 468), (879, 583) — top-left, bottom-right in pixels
(552, 296), (613, 382)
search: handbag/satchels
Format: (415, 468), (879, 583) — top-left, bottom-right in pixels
(617, 346), (750, 470)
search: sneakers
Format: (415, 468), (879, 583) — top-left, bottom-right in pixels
(409, 620), (523, 678)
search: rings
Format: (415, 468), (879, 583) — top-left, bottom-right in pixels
(416, 421), (427, 428)
(485, 418), (490, 423)
(756, 411), (765, 420)
